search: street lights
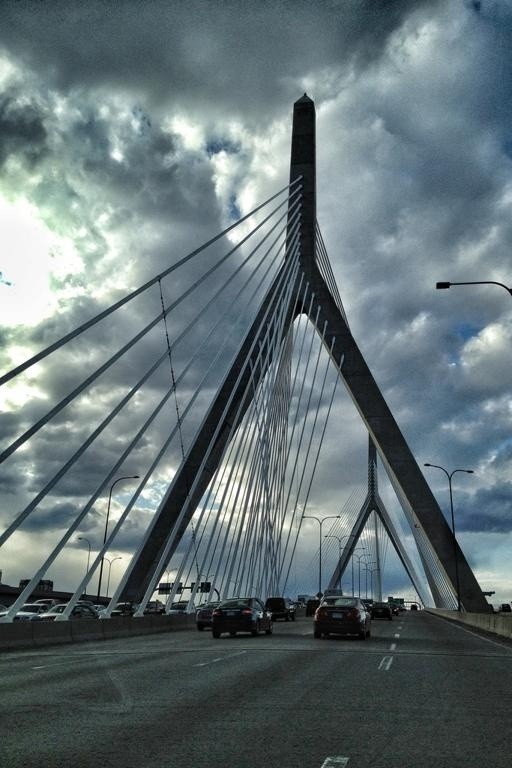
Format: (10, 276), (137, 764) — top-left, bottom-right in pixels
(325, 533), (353, 595)
(302, 515), (341, 595)
(433, 279), (512, 296)
(77, 538), (91, 595)
(198, 574), (215, 606)
(340, 545), (381, 599)
(164, 568), (181, 608)
(423, 463), (474, 612)
(97, 475), (141, 597)
